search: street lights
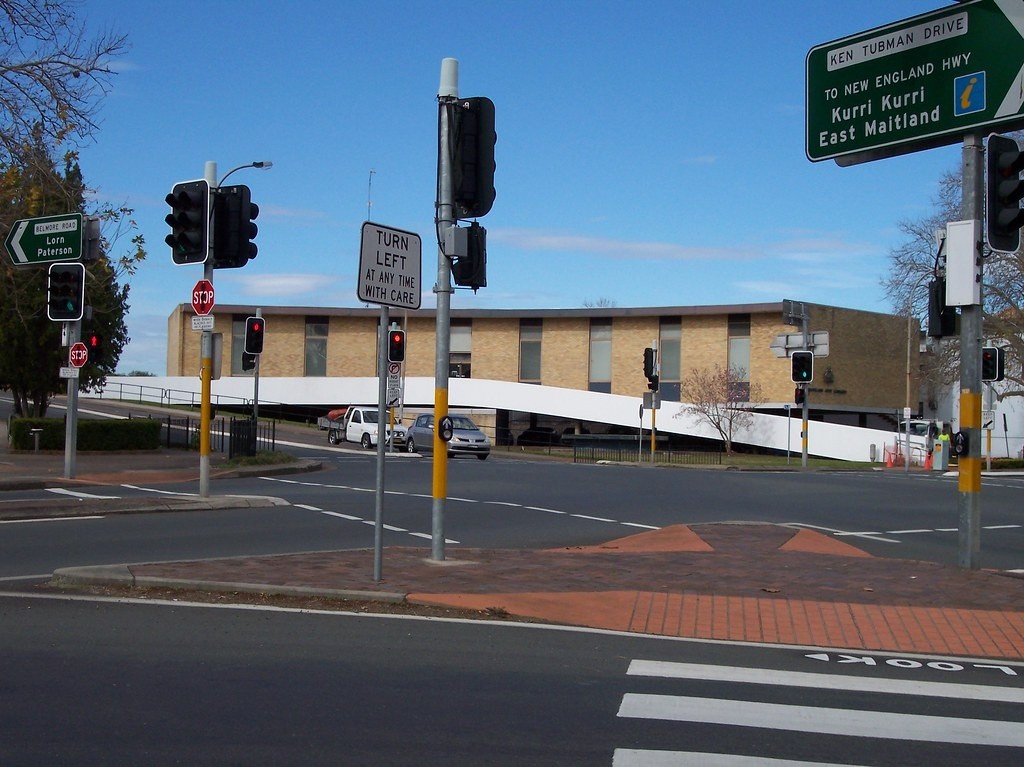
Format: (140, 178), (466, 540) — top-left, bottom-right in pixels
(199, 160), (273, 498)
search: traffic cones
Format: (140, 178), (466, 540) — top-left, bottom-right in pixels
(921, 455), (932, 472)
(885, 454), (894, 468)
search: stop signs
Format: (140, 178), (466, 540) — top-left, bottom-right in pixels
(191, 280), (216, 316)
(69, 342), (88, 367)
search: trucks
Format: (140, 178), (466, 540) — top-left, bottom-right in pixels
(901, 420), (954, 456)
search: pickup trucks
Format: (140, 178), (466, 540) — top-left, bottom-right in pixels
(318, 405), (409, 452)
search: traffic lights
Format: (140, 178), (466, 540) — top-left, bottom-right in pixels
(48, 262), (85, 321)
(216, 184), (258, 268)
(244, 317), (264, 354)
(642, 348), (653, 377)
(795, 389), (803, 404)
(89, 333), (102, 363)
(987, 133), (1024, 254)
(792, 351), (813, 383)
(982, 347), (998, 381)
(648, 375), (658, 391)
(164, 179), (209, 266)
(388, 330), (406, 363)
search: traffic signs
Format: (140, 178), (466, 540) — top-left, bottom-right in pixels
(981, 411), (994, 430)
(4, 213), (83, 266)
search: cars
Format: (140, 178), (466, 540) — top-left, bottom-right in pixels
(407, 413), (491, 460)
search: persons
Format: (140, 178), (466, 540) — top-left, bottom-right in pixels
(939, 430), (951, 449)
